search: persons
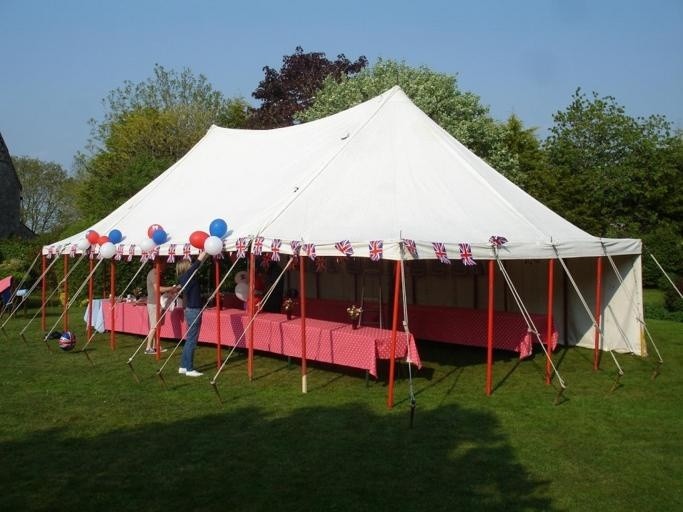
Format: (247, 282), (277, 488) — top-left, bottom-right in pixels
(141, 257), (178, 355)
(174, 249), (206, 377)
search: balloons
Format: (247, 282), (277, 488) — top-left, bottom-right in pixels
(147, 224), (162, 239)
(234, 281), (248, 303)
(188, 230), (208, 249)
(152, 230), (165, 245)
(209, 218), (226, 238)
(108, 230), (122, 244)
(233, 270), (248, 285)
(85, 230), (98, 244)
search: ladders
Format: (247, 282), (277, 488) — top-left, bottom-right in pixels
(358, 270), (382, 330)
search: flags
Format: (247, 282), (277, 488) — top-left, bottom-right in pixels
(487, 235), (508, 245)
(368, 239), (382, 263)
(400, 239), (418, 260)
(42, 236), (317, 263)
(334, 239), (353, 258)
(458, 242), (476, 268)
(432, 241), (451, 265)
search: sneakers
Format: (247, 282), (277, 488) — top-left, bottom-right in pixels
(179, 368), (204, 377)
(144, 346), (167, 355)
